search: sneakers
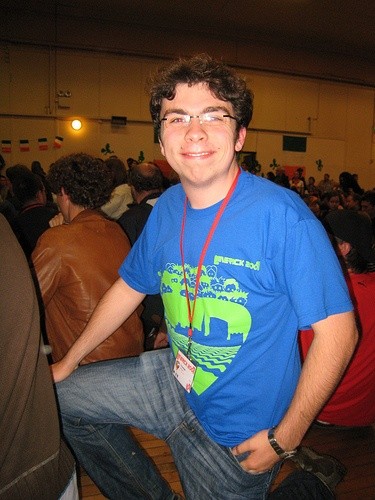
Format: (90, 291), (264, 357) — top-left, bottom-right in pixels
(287, 445), (348, 494)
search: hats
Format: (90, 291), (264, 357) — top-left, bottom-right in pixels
(321, 210), (371, 241)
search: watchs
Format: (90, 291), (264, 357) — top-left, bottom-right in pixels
(266, 424), (299, 462)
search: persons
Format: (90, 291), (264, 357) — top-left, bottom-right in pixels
(51, 53), (361, 500)
(0, 150), (374, 219)
(28, 152), (147, 365)
(9, 172), (60, 255)
(0, 211), (80, 500)
(115, 162), (169, 246)
(298, 207), (375, 431)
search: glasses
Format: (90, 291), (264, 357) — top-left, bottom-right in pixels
(159, 112), (237, 128)
